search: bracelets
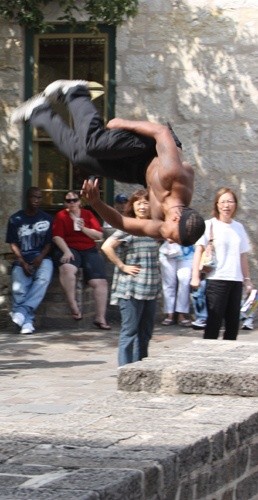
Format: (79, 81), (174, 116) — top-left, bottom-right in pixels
(244, 277), (250, 280)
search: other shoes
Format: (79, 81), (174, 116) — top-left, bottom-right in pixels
(176, 318), (191, 327)
(161, 318), (173, 325)
(20, 322), (37, 334)
(8, 311), (25, 327)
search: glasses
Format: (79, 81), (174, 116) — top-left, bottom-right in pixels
(216, 200), (235, 206)
(116, 201), (129, 204)
(64, 198), (79, 203)
(28, 194), (44, 202)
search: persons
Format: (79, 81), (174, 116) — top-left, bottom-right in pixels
(159, 240), (195, 326)
(6, 187), (54, 333)
(102, 194), (130, 238)
(190, 187), (253, 340)
(101, 189), (162, 365)
(50, 190), (111, 329)
(12, 80), (206, 246)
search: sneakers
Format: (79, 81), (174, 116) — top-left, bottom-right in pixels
(241, 317), (255, 329)
(10, 92), (50, 123)
(41, 79), (89, 103)
(191, 318), (206, 327)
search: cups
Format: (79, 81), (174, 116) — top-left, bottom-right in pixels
(74, 218), (82, 231)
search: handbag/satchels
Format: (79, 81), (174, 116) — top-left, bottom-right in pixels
(158, 241), (182, 257)
(198, 219), (216, 273)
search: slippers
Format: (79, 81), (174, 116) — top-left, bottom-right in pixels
(93, 320), (111, 330)
(72, 305), (83, 320)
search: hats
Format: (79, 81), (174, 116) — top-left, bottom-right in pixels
(115, 194), (129, 203)
(180, 206), (206, 247)
